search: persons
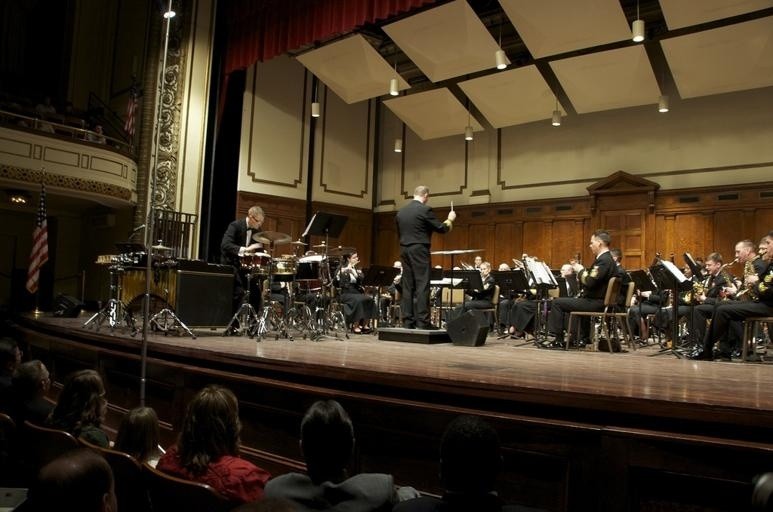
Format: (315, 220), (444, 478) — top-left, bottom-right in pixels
(618, 252), (734, 357)
(392, 414), (546, 512)
(10, 97), (111, 145)
(394, 185), (456, 330)
(220, 206), (266, 334)
(263, 398), (423, 512)
(687, 234), (772, 362)
(465, 254), (583, 338)
(375, 260), (403, 321)
(233, 496), (298, 511)
(537, 229), (633, 349)
(155, 383), (274, 504)
(268, 249), (375, 334)
(0, 339), (166, 512)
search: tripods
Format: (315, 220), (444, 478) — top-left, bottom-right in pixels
(83, 262), (135, 334)
(512, 284), (548, 348)
(646, 279), (682, 360)
(129, 239), (199, 339)
(223, 240), (351, 343)
(496, 288), (520, 341)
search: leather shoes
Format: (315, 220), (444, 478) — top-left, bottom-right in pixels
(353, 326), (377, 334)
(541, 337), (591, 350)
(418, 324), (440, 330)
(685, 337), (767, 361)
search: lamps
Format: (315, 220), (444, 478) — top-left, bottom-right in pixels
(394, 140), (403, 154)
(390, 64), (399, 96)
(658, 93), (669, 113)
(632, 4), (645, 43)
(465, 104), (474, 141)
(495, 38), (507, 69)
(312, 85), (320, 117)
(551, 89), (561, 127)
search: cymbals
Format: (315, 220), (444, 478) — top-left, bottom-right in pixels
(329, 246), (357, 255)
(289, 241), (307, 246)
(313, 244), (332, 248)
(252, 230), (292, 245)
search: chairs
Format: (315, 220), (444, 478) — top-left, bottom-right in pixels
(262, 278), (773, 363)
(0, 381), (443, 512)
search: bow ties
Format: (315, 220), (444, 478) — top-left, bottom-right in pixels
(246, 227), (255, 231)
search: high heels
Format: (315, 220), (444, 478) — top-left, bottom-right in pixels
(511, 331), (525, 339)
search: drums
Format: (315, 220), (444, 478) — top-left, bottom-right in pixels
(298, 254), (326, 291)
(295, 261), (319, 283)
(271, 257), (297, 275)
(238, 250), (270, 277)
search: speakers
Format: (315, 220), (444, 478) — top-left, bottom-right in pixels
(444, 309), (489, 347)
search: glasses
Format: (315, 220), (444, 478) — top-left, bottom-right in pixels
(251, 215), (264, 225)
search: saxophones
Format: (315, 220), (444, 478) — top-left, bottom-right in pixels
(684, 250), (766, 303)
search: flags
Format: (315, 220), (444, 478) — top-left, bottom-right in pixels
(124, 84), (138, 135)
(26, 175), (50, 293)
